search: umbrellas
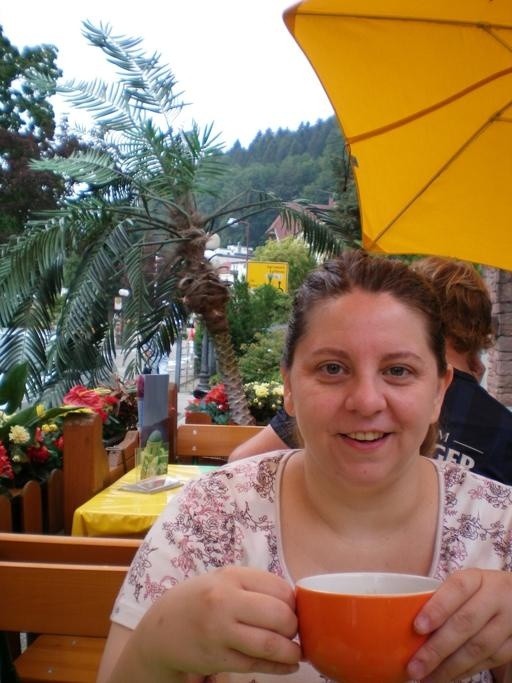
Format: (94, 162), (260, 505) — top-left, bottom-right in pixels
(277, 0), (512, 273)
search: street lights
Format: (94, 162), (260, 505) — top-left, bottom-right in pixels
(198, 218), (251, 392)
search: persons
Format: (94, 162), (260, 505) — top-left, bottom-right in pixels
(91, 246), (512, 682)
(226, 254), (512, 487)
(191, 388), (209, 411)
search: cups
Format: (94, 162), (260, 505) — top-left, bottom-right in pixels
(288, 574), (440, 683)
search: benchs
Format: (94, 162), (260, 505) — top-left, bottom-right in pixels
(2, 532), (143, 681)
(176, 424), (266, 465)
(1, 561), (131, 683)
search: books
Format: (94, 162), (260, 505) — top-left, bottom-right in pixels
(120, 475), (184, 493)
